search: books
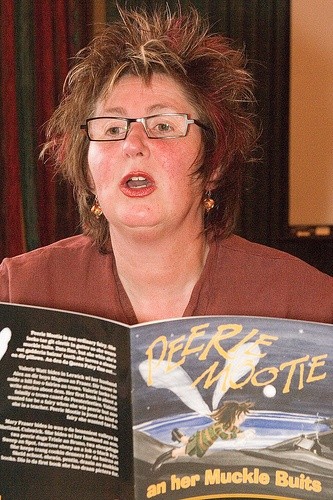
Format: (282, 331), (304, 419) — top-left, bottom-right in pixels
(0, 302), (333, 500)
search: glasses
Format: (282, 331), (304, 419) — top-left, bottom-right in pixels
(80, 113), (213, 141)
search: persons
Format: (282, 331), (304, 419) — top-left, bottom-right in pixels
(0, 2), (333, 326)
